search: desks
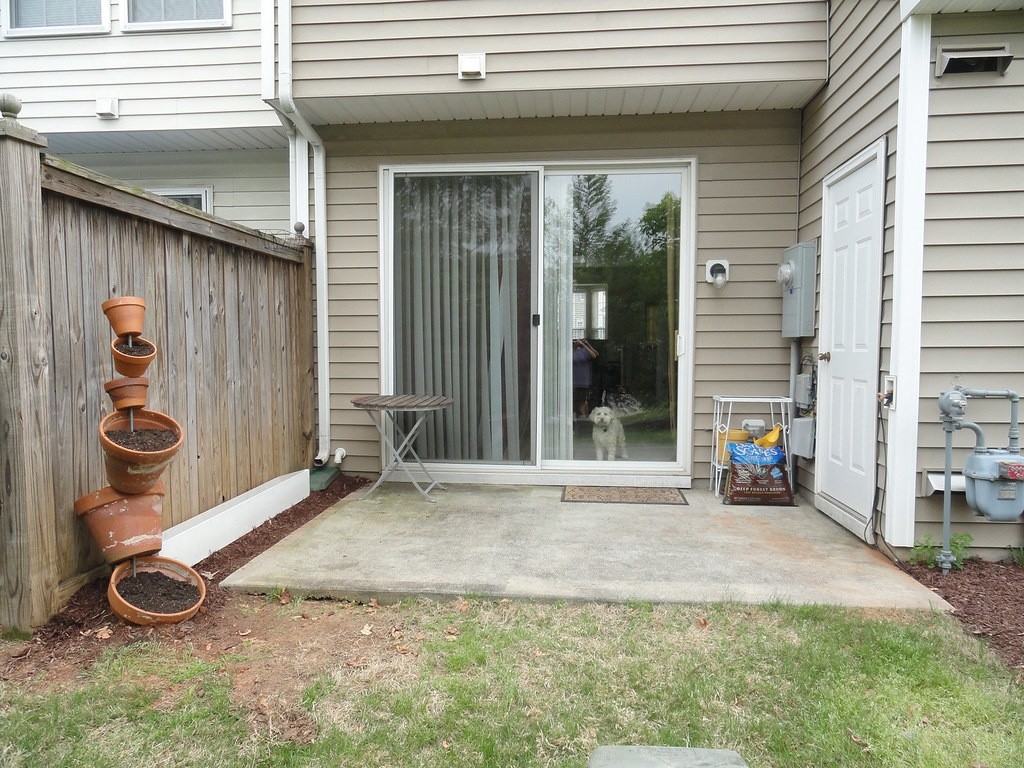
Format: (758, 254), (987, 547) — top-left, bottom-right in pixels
(709, 394), (793, 498)
(351, 397), (453, 498)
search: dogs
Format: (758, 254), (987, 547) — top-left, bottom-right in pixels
(588, 406), (628, 461)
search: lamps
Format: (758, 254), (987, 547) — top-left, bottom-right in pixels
(457, 53), (486, 79)
(705, 260), (729, 288)
(96, 99), (119, 120)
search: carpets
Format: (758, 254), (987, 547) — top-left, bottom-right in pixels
(560, 485), (689, 505)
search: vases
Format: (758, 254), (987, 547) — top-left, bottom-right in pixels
(99, 411), (184, 493)
(74, 480), (164, 567)
(111, 337), (157, 378)
(104, 378), (149, 411)
(109, 556), (206, 625)
(102, 298), (146, 337)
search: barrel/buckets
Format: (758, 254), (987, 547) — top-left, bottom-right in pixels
(718, 430), (748, 464)
